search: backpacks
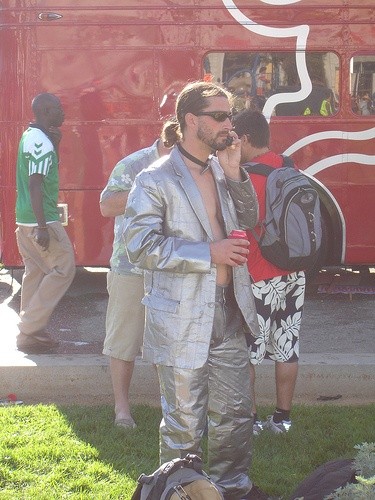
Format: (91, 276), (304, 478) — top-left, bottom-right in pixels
(130, 454), (225, 500)
(240, 154), (328, 272)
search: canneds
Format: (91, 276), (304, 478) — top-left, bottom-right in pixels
(228, 230), (248, 263)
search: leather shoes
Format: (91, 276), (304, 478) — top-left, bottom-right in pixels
(20, 331), (60, 346)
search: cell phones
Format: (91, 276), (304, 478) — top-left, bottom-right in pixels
(227, 136), (233, 146)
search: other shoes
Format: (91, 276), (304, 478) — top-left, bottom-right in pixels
(236, 483), (274, 500)
(266, 415), (291, 435)
(252, 420), (264, 436)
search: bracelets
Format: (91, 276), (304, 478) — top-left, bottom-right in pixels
(37, 226), (48, 229)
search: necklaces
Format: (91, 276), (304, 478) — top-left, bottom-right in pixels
(175, 142), (211, 175)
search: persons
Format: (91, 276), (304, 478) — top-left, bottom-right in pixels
(15, 89), (78, 350)
(319, 94), (334, 116)
(255, 65), (271, 95)
(121, 80), (274, 500)
(231, 108), (307, 437)
(99, 138), (172, 435)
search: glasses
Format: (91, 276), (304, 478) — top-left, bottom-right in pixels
(193, 111), (233, 122)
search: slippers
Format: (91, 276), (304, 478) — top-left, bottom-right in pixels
(116, 418), (137, 432)
